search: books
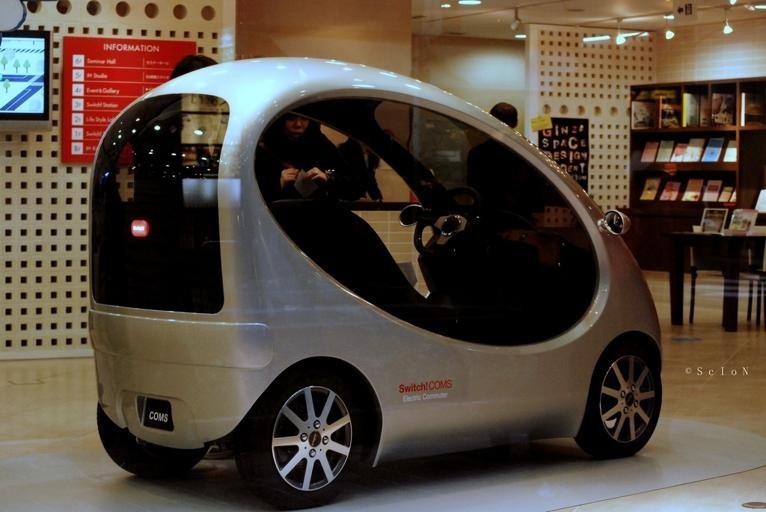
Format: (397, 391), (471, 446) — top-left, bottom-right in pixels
(754, 189), (766, 213)
(629, 87), (764, 202)
(700, 207), (729, 235)
(728, 207), (758, 236)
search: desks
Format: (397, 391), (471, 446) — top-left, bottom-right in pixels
(667, 227), (765, 333)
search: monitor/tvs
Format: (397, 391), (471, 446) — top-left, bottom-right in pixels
(0, 30), (52, 126)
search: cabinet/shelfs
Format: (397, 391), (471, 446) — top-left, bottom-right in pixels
(630, 75), (766, 272)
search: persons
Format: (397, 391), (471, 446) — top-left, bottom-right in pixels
(254, 113), (365, 205)
(466, 101), (547, 215)
(168, 53), (217, 80)
(333, 134), (383, 204)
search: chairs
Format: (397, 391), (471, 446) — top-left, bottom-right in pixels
(266, 171), (463, 340)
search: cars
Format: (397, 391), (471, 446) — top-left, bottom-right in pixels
(83, 47), (666, 511)
